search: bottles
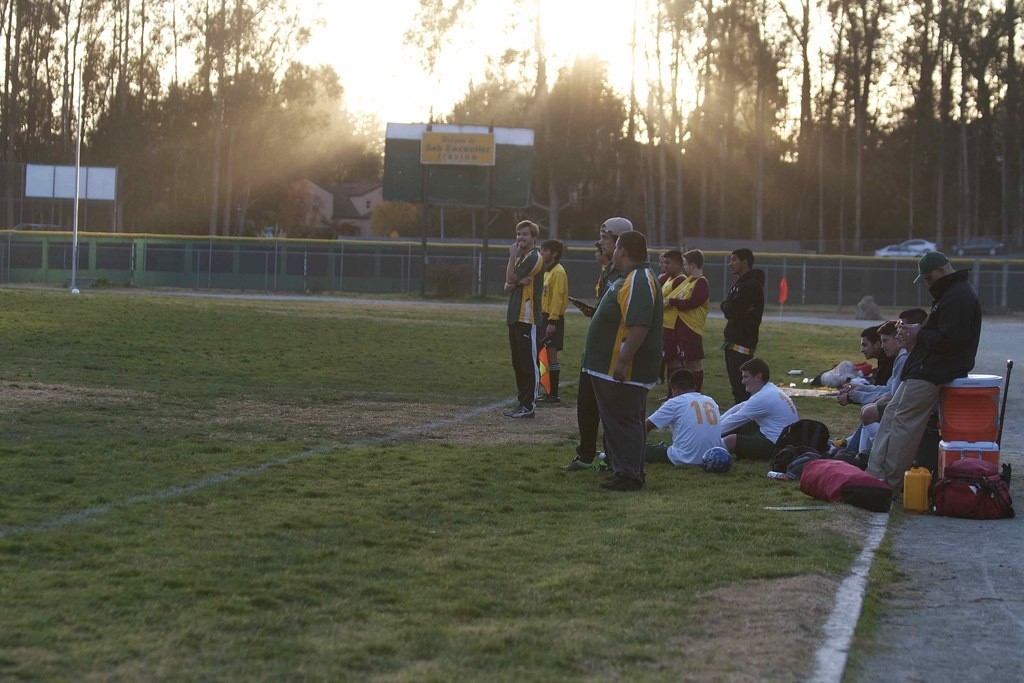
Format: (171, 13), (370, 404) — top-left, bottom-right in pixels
(767, 471), (790, 482)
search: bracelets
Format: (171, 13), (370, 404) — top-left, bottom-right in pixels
(847, 392), (852, 403)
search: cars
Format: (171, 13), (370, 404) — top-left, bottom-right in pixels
(950, 236), (1007, 257)
(899, 239), (938, 254)
(875, 245), (924, 258)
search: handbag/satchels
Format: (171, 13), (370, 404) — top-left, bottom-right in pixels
(930, 476), (1015, 519)
(770, 444), (894, 511)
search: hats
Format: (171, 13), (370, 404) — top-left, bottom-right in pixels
(912, 251), (945, 283)
(600, 217), (633, 236)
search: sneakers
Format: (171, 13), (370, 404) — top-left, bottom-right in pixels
(502, 404), (537, 418)
(599, 457), (612, 471)
(600, 470), (647, 491)
(536, 393), (560, 402)
(656, 395), (669, 404)
(564, 455), (597, 470)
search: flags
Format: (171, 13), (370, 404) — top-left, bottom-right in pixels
(778, 278), (788, 301)
(538, 343), (550, 394)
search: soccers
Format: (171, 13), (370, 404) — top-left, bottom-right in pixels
(701, 447), (732, 475)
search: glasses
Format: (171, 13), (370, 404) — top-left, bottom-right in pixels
(897, 319), (920, 326)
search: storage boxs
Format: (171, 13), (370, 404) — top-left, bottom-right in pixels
(940, 373), (1003, 440)
(939, 441), (999, 478)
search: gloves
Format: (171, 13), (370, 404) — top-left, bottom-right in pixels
(836, 392), (849, 406)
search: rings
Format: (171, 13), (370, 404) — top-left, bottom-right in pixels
(908, 332), (910, 336)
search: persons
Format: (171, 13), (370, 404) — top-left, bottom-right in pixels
(504, 219), (545, 418)
(561, 216), (981, 501)
(535, 239), (568, 403)
